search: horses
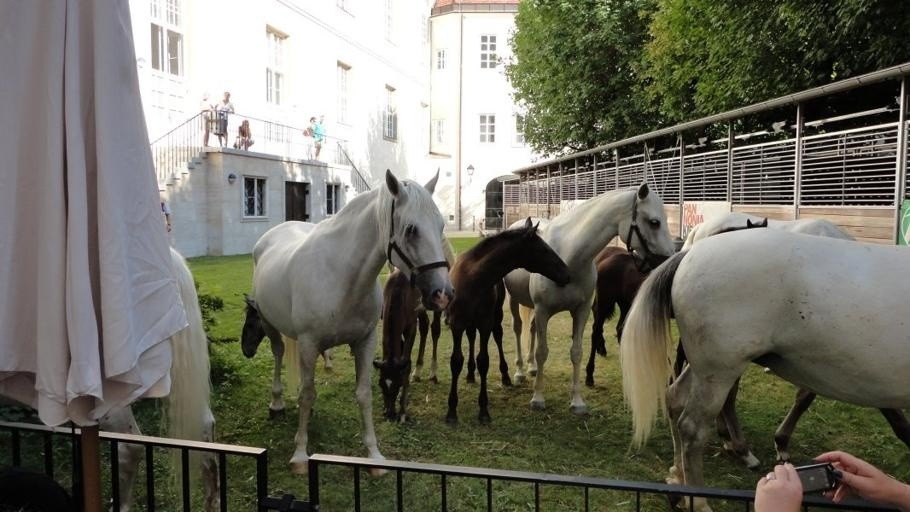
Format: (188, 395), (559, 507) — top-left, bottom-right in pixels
(98, 244), (221, 511)
(241, 167), (909, 512)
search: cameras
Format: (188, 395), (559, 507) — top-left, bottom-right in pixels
(794, 461), (837, 494)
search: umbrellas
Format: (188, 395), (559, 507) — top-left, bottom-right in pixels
(0, 1), (195, 509)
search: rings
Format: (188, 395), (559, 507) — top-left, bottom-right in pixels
(765, 470), (776, 481)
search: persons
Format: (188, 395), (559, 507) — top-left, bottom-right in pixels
(235, 120), (255, 151)
(202, 96), (213, 148)
(752, 446), (910, 512)
(307, 115), (325, 158)
(214, 91), (234, 148)
